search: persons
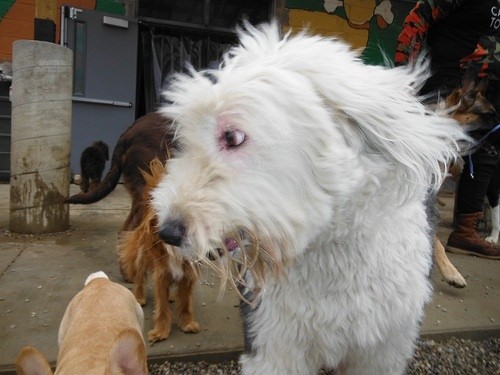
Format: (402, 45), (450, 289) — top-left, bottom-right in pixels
(407, 0), (500, 262)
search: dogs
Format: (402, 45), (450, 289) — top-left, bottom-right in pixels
(114, 157), (201, 345)
(60, 107), (469, 289)
(15, 269), (150, 375)
(79, 139), (111, 193)
(143, 15), (479, 375)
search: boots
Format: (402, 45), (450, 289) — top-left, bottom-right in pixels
(443, 212), (500, 260)
